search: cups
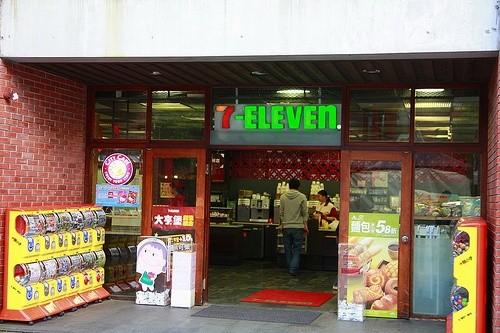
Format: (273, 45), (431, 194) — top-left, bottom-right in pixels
(276, 181), (290, 195)
(251, 192), (270, 200)
(388, 244), (399, 260)
(322, 220), (328, 229)
(329, 194), (340, 203)
(310, 180), (325, 195)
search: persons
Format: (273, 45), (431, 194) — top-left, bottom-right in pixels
(168, 180), (186, 207)
(279, 180), (309, 277)
(313, 189), (338, 229)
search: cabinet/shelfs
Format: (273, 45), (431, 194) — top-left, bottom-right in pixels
(0, 204), (113, 323)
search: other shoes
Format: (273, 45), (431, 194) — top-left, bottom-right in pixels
(289, 271), (296, 275)
(333, 283), (338, 290)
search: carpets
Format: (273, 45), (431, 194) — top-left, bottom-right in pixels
(191, 304), (322, 326)
(241, 289), (336, 307)
